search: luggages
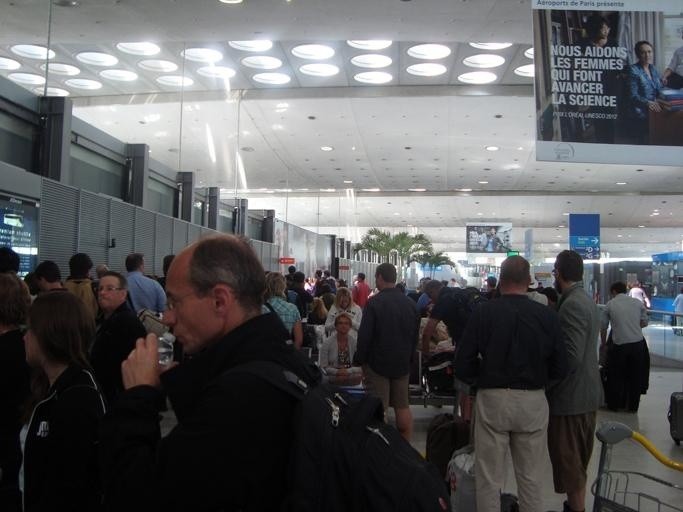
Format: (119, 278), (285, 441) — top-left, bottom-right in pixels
(668, 392), (683, 444)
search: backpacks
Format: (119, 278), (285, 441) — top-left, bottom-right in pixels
(455, 287), (489, 320)
(218, 358), (452, 512)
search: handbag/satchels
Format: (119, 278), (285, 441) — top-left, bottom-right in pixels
(138, 309), (170, 337)
(426, 412), (477, 512)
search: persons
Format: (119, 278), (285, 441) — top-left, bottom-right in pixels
(2, 233), (682, 511)
(565, 14), (683, 146)
(469, 226), (503, 252)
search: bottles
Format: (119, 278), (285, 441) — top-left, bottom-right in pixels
(154, 332), (174, 367)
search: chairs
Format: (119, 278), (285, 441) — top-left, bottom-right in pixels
(300, 323), (462, 407)
